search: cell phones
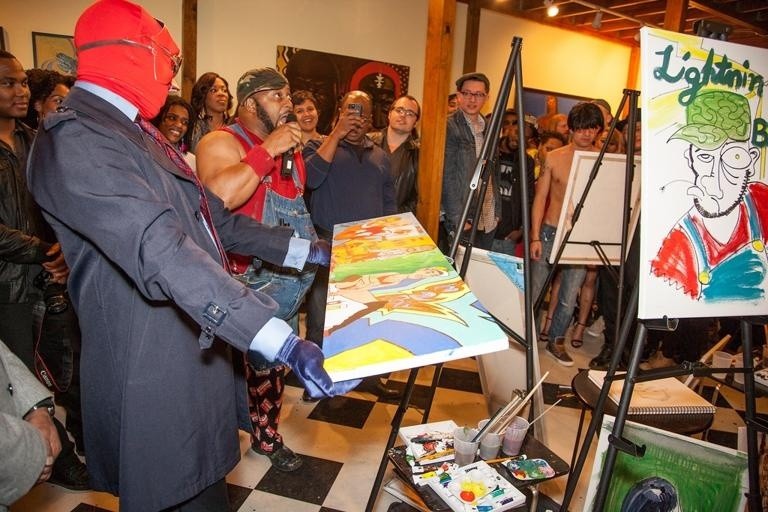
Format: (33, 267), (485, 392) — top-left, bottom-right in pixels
(349, 104), (362, 116)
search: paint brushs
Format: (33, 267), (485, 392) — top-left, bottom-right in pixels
(471, 371), (562, 442)
(415, 448), (454, 462)
(484, 455), (520, 464)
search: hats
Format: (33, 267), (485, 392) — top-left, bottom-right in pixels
(74, 0), (180, 120)
(235, 67), (289, 106)
(592, 98), (612, 114)
(455, 72), (490, 87)
(503, 120), (517, 126)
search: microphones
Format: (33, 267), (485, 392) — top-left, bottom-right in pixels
(281, 114), (297, 176)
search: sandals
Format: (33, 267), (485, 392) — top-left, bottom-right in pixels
(540, 316), (551, 341)
(571, 322), (585, 348)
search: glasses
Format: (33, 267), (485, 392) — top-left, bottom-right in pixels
(391, 106), (417, 118)
(150, 38), (182, 76)
(459, 90), (485, 99)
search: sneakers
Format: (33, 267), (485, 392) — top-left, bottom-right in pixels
(587, 316), (605, 337)
(45, 442), (93, 493)
(545, 336), (575, 366)
(251, 443), (303, 472)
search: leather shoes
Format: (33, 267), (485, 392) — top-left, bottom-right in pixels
(355, 377), (401, 399)
(302, 386), (322, 403)
(638, 360), (653, 375)
(589, 346), (621, 370)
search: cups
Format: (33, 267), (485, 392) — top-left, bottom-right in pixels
(453, 426), (481, 467)
(478, 419), (505, 462)
(713, 350), (752, 384)
(500, 415), (530, 457)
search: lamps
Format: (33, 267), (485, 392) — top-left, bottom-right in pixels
(591, 1), (603, 30)
(541, 0), (560, 18)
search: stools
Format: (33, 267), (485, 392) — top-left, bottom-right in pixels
(560, 365), (717, 512)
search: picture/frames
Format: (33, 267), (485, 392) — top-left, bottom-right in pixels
(511, 84), (598, 120)
(31, 29), (79, 78)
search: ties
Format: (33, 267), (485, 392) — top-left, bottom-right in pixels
(138, 117), (232, 273)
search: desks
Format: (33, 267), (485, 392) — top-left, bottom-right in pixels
(708, 342), (766, 411)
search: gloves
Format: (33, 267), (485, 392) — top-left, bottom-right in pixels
(309, 235), (330, 265)
(276, 333), (364, 401)
